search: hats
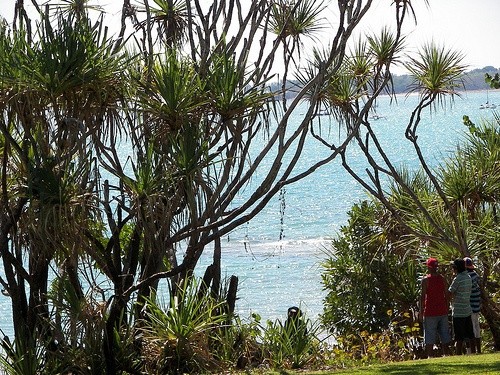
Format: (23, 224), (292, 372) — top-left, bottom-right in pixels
(426, 258), (438, 270)
(450, 259), (465, 273)
(463, 257), (477, 269)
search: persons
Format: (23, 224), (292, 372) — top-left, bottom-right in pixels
(447, 258), (476, 356)
(418, 258), (452, 358)
(463, 257), (482, 354)
